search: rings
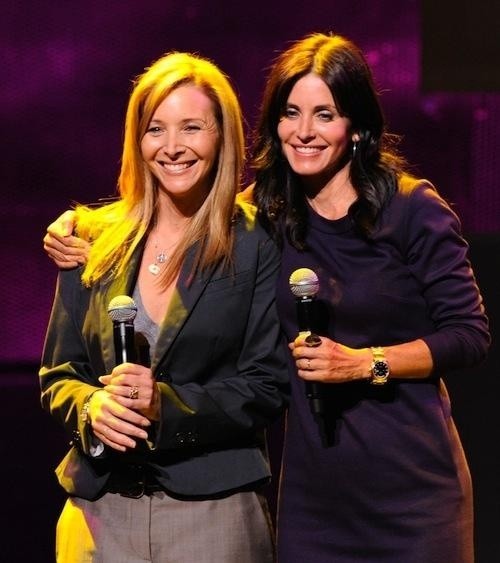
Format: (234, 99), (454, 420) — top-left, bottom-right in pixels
(305, 334), (322, 347)
(129, 386), (138, 399)
(307, 359), (311, 370)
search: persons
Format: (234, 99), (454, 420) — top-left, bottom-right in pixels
(38, 51), (291, 563)
(43, 32), (492, 563)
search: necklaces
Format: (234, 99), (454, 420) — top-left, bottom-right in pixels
(148, 231), (181, 275)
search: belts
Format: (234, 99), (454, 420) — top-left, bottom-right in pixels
(107, 466), (164, 499)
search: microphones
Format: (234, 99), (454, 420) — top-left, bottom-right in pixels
(108, 293), (139, 364)
(288, 268), (320, 414)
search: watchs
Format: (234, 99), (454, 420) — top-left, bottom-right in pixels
(368, 346), (390, 386)
(80, 398), (91, 425)
